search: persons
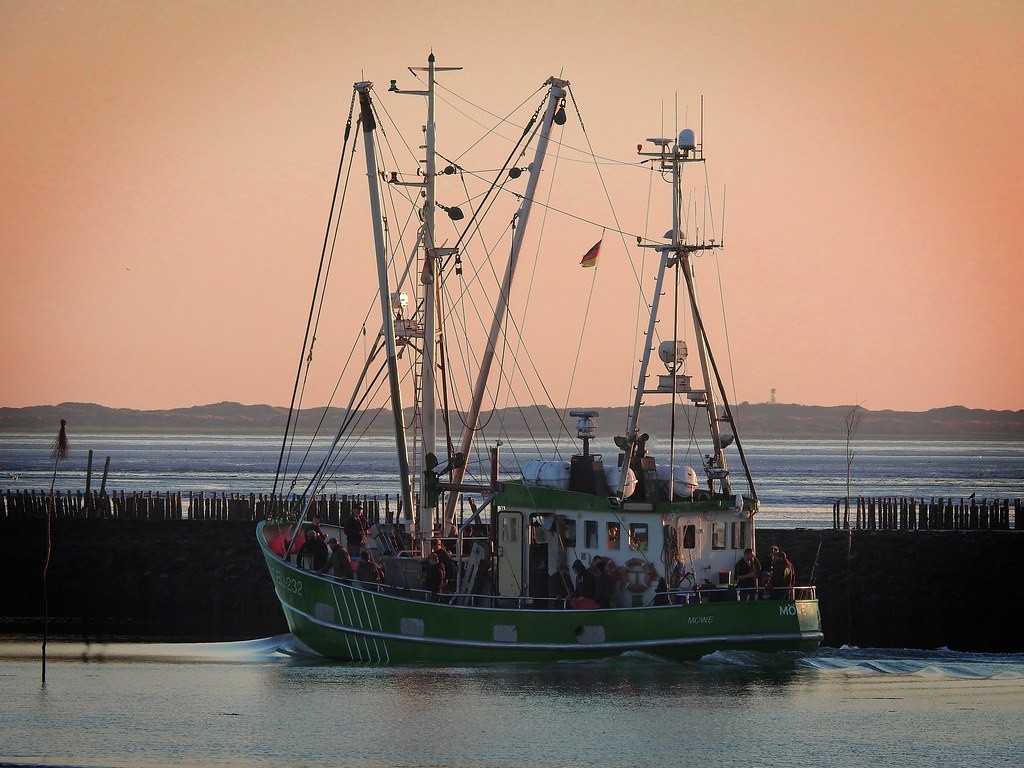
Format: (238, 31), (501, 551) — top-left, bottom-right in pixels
(428, 525), (477, 604)
(734, 546), (795, 602)
(296, 505), (384, 592)
(548, 556), (610, 611)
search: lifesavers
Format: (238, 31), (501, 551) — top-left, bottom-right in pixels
(619, 558), (653, 591)
(596, 555), (618, 583)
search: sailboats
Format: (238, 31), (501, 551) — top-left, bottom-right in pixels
(255, 45), (829, 663)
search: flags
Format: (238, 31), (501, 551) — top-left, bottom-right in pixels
(579, 240), (602, 268)
